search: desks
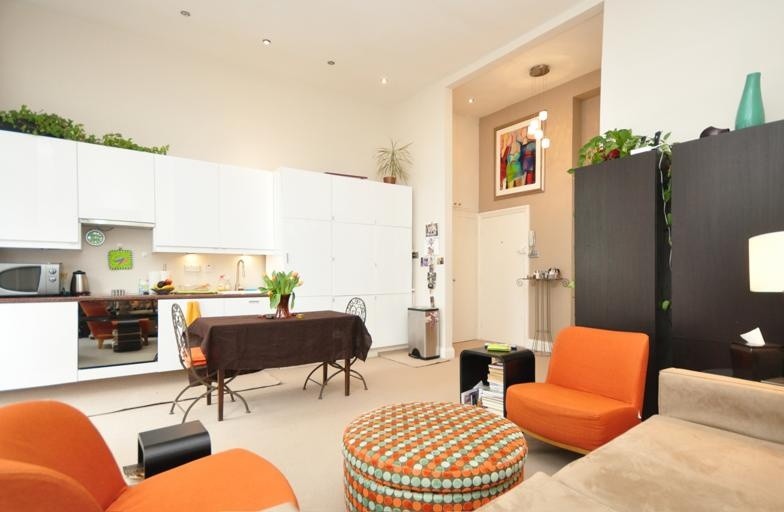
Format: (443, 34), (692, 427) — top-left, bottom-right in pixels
(515, 277), (571, 358)
(458, 344), (536, 418)
(180, 309), (372, 422)
(127, 308), (158, 337)
(698, 366), (783, 386)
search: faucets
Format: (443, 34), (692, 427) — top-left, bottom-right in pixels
(235, 259), (247, 290)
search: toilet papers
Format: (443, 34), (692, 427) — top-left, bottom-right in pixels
(160, 271), (169, 279)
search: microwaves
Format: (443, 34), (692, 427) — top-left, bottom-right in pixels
(0, 262), (62, 296)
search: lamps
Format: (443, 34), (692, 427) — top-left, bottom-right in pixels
(525, 62), (554, 148)
(746, 229), (783, 294)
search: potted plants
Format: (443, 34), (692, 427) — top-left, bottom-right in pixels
(566, 130), (681, 314)
(373, 136), (416, 186)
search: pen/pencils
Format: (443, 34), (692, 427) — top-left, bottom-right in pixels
(484, 342), (517, 352)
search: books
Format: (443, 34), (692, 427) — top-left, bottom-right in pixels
(484, 342), (517, 351)
(461, 356), (503, 416)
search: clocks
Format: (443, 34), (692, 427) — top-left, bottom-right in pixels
(84, 229), (105, 246)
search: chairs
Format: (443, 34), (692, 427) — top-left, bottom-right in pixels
(168, 303), (251, 424)
(79, 301), (148, 350)
(0, 396), (301, 511)
(301, 296), (369, 399)
(504, 323), (652, 455)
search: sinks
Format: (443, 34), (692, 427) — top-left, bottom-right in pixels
(218, 290), (261, 294)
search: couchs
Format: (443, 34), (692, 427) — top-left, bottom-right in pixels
(474, 367), (783, 512)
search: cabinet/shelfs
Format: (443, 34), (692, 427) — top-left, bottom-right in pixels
(333, 224), (412, 295)
(154, 154), (278, 255)
(0, 128), (84, 250)
(274, 165), (333, 222)
(333, 175), (413, 229)
(76, 141), (154, 230)
(266, 222), (332, 292)
(292, 293), (334, 312)
(334, 292), (413, 350)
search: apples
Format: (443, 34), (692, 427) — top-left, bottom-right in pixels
(165, 279), (173, 284)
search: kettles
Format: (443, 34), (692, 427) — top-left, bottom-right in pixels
(70, 269), (90, 295)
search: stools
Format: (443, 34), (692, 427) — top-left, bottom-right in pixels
(341, 398), (529, 512)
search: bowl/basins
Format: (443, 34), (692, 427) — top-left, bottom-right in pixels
(149, 287), (175, 294)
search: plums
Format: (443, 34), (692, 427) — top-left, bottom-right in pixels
(158, 281), (165, 288)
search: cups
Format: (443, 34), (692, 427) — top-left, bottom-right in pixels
(111, 289), (126, 296)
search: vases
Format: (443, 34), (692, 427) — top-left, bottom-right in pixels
(274, 293), (293, 319)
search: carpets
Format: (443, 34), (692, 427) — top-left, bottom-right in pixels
(377, 347), (451, 369)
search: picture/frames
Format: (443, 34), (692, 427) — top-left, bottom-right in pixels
(492, 108), (545, 203)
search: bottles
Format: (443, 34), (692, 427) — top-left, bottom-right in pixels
(160, 263), (168, 281)
(184, 281), (193, 290)
(216, 275), (232, 291)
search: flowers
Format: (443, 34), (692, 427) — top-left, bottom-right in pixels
(253, 268), (305, 310)
